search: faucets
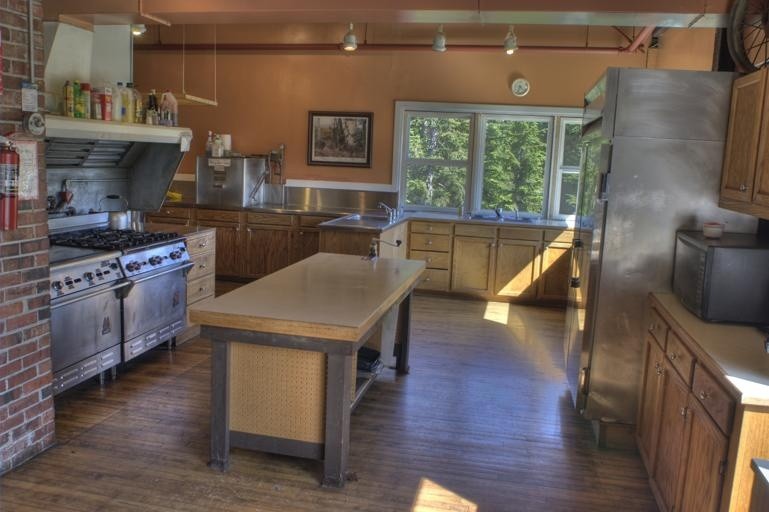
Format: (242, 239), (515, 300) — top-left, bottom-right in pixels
(376, 202), (396, 220)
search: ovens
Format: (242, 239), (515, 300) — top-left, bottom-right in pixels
(117, 242), (194, 363)
(50, 260), (133, 397)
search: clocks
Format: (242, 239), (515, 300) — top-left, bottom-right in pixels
(511, 77), (530, 98)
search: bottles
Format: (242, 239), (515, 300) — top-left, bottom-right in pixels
(62, 79), (173, 127)
(205, 130), (213, 154)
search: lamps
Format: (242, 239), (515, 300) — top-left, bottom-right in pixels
(504, 22), (518, 55)
(130, 24), (148, 36)
(432, 22), (447, 53)
(342, 21), (358, 52)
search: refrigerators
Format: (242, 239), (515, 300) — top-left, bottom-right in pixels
(564, 67), (760, 424)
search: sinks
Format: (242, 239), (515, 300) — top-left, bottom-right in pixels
(347, 215), (389, 222)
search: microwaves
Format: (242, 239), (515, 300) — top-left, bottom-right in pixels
(671, 230), (769, 323)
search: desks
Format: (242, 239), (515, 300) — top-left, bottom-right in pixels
(190, 250), (430, 489)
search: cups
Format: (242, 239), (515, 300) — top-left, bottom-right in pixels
(703, 222), (724, 238)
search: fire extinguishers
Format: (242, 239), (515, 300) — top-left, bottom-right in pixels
(0, 131), (20, 231)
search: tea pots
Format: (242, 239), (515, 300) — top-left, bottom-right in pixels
(99, 195), (131, 231)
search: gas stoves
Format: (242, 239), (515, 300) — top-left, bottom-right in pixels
(48, 228), (186, 255)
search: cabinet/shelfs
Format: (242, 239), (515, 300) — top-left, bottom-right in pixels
(185, 229), (217, 325)
(197, 210), (295, 279)
(453, 223), (545, 299)
(143, 206), (193, 226)
(299, 215), (338, 260)
(718, 68), (768, 221)
(637, 302), (670, 475)
(538, 227), (574, 301)
(651, 331), (734, 512)
(408, 223), (454, 292)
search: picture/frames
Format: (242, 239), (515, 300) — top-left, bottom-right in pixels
(306, 110), (373, 169)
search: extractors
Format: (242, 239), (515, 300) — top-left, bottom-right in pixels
(44, 115), (193, 213)
